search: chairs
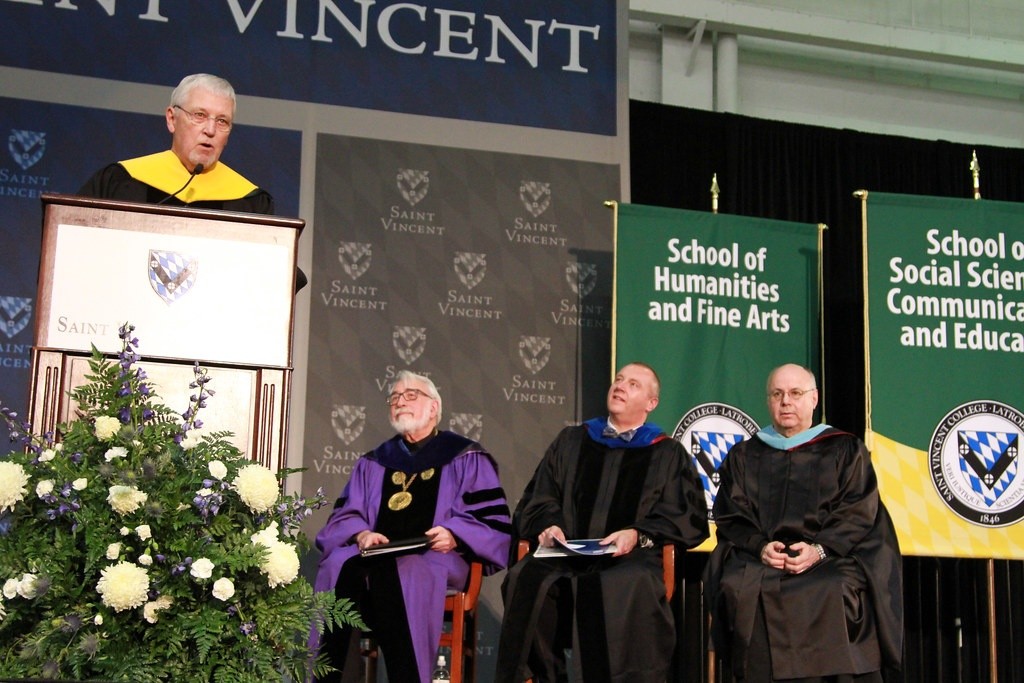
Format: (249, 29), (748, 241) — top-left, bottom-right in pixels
(432, 560), (489, 683)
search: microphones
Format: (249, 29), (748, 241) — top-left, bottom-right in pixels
(156, 164), (204, 205)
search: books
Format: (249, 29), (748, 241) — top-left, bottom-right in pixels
(361, 537), (429, 561)
(533, 532), (618, 559)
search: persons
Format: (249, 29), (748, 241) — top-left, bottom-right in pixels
(308, 371), (511, 683)
(496, 361), (713, 683)
(73, 71), (308, 294)
(704, 360), (905, 683)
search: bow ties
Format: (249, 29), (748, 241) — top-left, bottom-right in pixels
(602, 426), (637, 443)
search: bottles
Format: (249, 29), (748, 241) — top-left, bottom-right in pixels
(432, 655), (451, 683)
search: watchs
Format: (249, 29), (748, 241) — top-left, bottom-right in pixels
(637, 530), (648, 547)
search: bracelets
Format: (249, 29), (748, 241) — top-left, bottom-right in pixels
(815, 543), (827, 560)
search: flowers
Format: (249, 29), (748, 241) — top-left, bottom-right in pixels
(0, 325), (369, 683)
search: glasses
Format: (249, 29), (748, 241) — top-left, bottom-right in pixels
(769, 389), (813, 403)
(385, 388), (434, 406)
(173, 105), (233, 132)
